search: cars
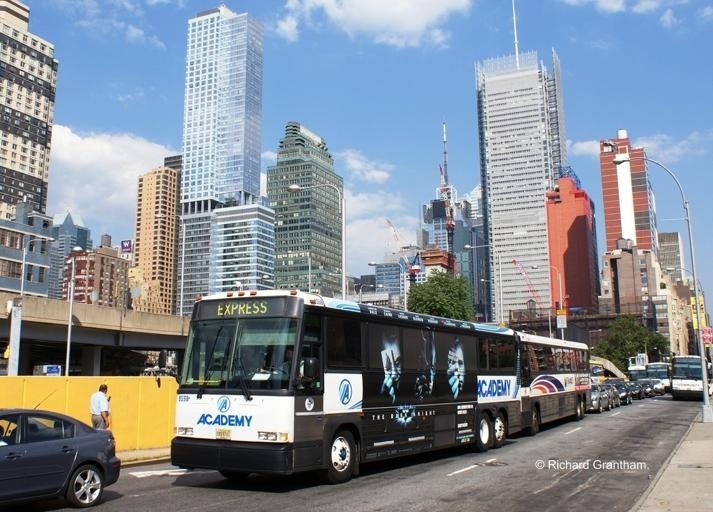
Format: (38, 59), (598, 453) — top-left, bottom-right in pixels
(0, 388), (122, 508)
(587, 376), (667, 413)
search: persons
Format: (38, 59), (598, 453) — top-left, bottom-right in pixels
(446, 339), (465, 399)
(0, 425), (7, 446)
(411, 326), (437, 400)
(378, 332), (403, 404)
(89, 384), (110, 430)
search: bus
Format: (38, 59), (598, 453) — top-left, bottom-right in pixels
(627, 354), (713, 402)
(166, 291), (522, 485)
(514, 331), (590, 440)
(589, 359), (605, 386)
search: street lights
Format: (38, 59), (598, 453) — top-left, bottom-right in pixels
(480, 278), (500, 324)
(155, 212), (186, 318)
(64, 244), (87, 375)
(666, 266), (713, 363)
(287, 182), (349, 303)
(368, 260), (405, 310)
(360, 284), (375, 305)
(532, 264), (565, 341)
(614, 155), (713, 424)
(462, 244), (505, 328)
(21, 237), (56, 297)
